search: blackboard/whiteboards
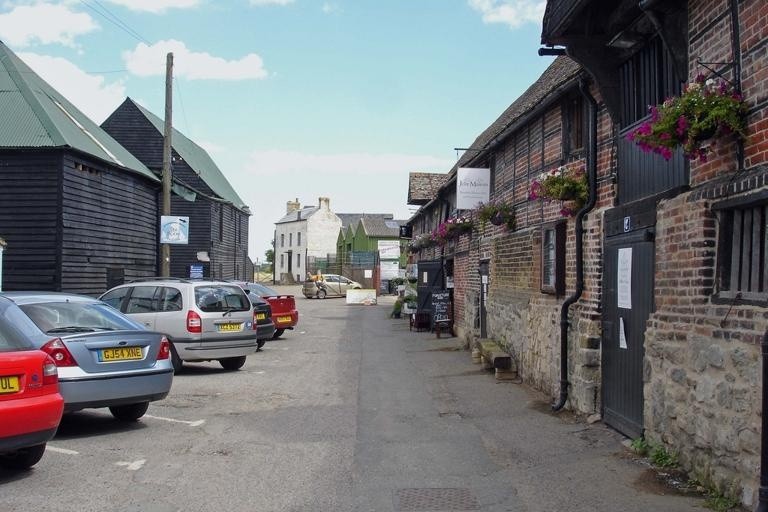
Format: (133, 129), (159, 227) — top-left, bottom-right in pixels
(430, 290), (452, 328)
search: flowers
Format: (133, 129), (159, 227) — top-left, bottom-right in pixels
(406, 215), (473, 253)
(528, 164), (592, 218)
(478, 199), (517, 232)
(624, 72), (749, 166)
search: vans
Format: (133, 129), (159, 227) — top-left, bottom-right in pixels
(301, 274), (362, 299)
(95, 275), (259, 371)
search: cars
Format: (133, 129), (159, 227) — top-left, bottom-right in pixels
(217, 290), (276, 351)
(0, 313), (66, 470)
(224, 279), (298, 338)
(0, 289), (176, 421)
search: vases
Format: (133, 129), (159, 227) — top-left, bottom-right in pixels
(563, 198), (581, 214)
(697, 110), (718, 142)
(489, 216), (503, 226)
